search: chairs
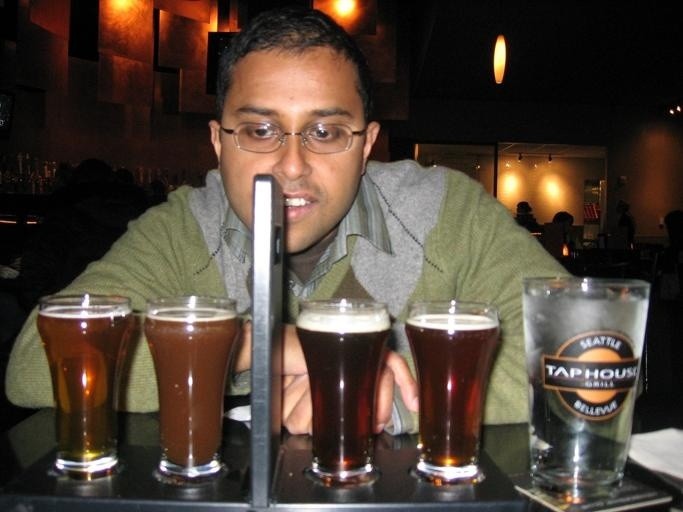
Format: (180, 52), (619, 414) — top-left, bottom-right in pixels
(545, 238), (664, 348)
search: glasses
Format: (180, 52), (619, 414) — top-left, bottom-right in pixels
(218, 121), (366, 155)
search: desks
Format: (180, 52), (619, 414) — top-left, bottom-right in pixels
(0, 403), (669, 512)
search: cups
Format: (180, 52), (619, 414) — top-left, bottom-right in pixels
(142, 293), (244, 487)
(126, 164), (178, 197)
(521, 278), (650, 493)
(35, 293), (136, 477)
(405, 300), (501, 482)
(296, 298), (392, 490)
(2, 153), (61, 197)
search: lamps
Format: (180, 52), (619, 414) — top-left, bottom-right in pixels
(516, 152), (524, 163)
(547, 153), (552, 164)
(492, 35), (506, 85)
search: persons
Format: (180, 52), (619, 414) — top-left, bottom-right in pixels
(2, 5), (643, 436)
(514, 197), (683, 303)
